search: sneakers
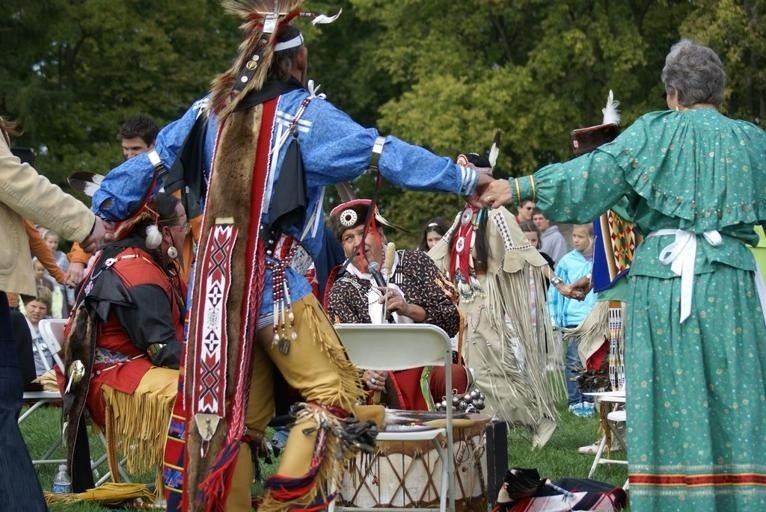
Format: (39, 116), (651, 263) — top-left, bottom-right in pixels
(583, 402), (594, 416)
(535, 417), (557, 449)
(568, 403), (583, 417)
(578, 438), (620, 455)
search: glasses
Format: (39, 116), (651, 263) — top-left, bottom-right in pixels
(168, 222), (192, 235)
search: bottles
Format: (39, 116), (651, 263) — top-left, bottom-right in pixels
(51, 463), (76, 493)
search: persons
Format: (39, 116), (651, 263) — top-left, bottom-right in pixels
(90, 2), (495, 511)
(22, 116), (651, 453)
(0, 109), (110, 512)
(474, 35), (765, 511)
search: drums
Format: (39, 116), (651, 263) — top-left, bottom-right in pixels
(338, 411), (489, 508)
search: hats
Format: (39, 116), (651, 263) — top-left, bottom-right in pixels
(569, 122), (620, 156)
(330, 199), (380, 240)
(273, 24), (304, 52)
(456, 153), (493, 176)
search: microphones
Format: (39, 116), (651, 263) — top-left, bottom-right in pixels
(368, 261), (398, 312)
